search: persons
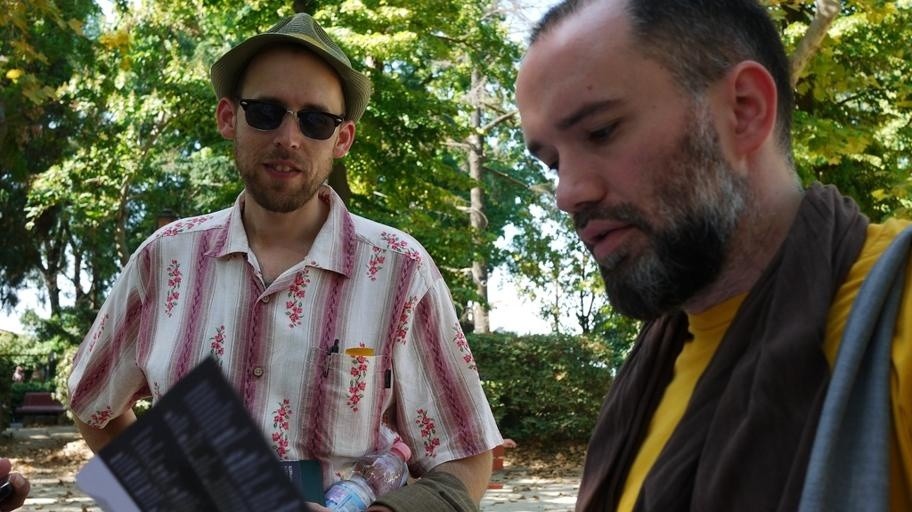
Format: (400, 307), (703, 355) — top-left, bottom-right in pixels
(0, 452), (32, 512)
(66, 0), (504, 512)
(515, 0), (912, 512)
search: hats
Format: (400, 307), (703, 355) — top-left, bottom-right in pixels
(210, 13), (370, 126)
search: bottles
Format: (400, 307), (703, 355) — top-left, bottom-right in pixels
(325, 440), (412, 511)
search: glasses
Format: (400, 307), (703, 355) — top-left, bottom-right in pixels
(235, 99), (345, 140)
